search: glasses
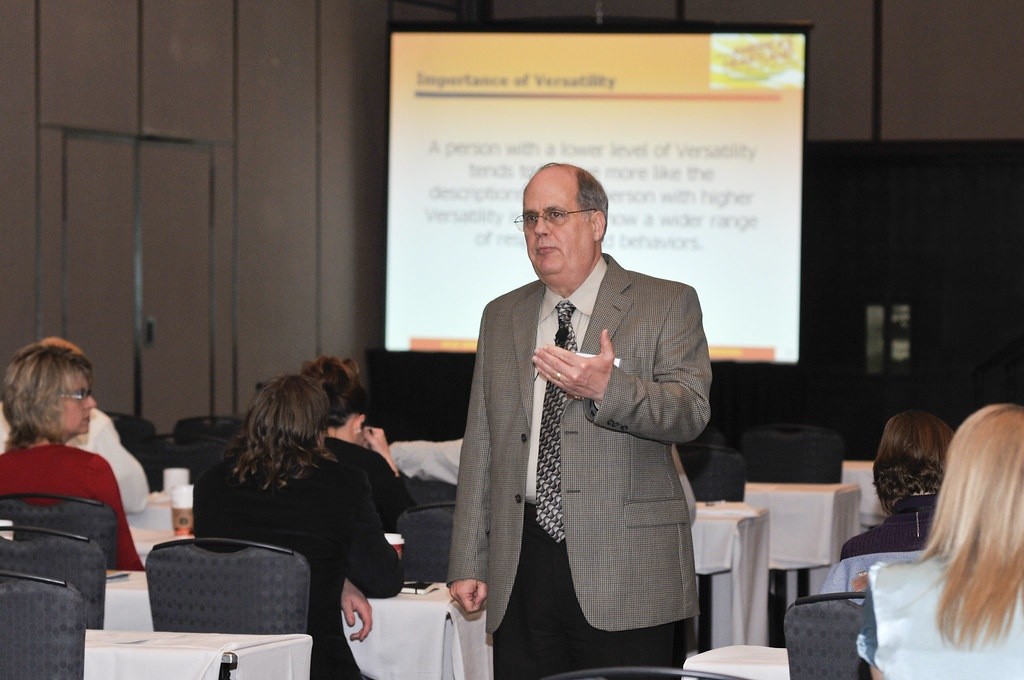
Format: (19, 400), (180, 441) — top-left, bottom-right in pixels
(513, 207), (596, 232)
(59, 387), (92, 400)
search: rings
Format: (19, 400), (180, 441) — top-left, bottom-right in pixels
(556, 371), (561, 378)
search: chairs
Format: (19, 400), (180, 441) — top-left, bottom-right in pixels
(0, 527), (106, 630)
(784, 592), (865, 680)
(144, 536), (310, 634)
(0, 495), (120, 573)
(676, 445), (745, 503)
(101, 411), (155, 441)
(397, 502), (456, 585)
(746, 423), (845, 483)
(127, 434), (221, 491)
(0, 570), (84, 679)
(173, 417), (245, 440)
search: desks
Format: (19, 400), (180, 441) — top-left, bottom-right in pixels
(745, 484), (864, 603)
(340, 584), (494, 678)
(683, 646), (801, 680)
(80, 622), (312, 679)
(694, 505), (771, 646)
(105, 571), (155, 632)
(840, 460), (888, 527)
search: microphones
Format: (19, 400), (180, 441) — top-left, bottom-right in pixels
(554, 328), (569, 342)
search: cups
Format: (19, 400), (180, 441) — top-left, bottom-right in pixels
(172, 485), (193, 537)
(163, 468), (190, 497)
(384, 532), (405, 559)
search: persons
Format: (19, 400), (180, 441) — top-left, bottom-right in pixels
(0, 337), (148, 571)
(192, 373), (404, 680)
(856, 403), (1024, 680)
(840, 411), (954, 562)
(299, 355), (417, 533)
(446, 163), (712, 680)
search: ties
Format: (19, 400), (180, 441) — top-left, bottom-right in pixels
(536, 300), (578, 543)
